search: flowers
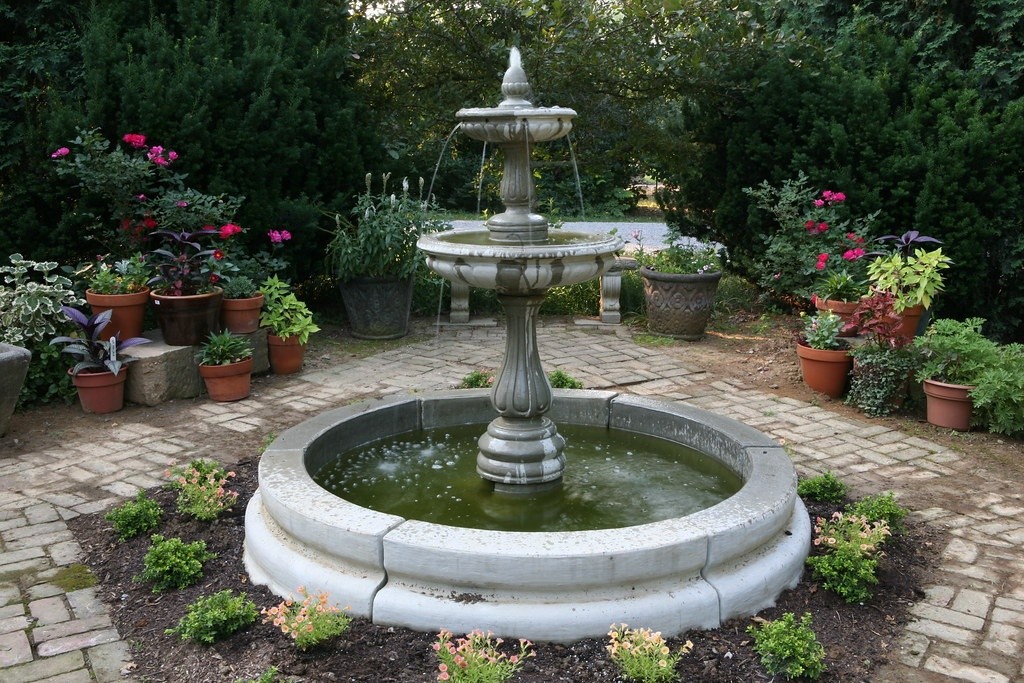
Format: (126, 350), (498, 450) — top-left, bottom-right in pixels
(632, 226), (722, 275)
(799, 305), (845, 350)
(0, 126), (321, 442)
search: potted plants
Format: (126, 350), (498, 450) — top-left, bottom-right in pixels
(867, 246), (954, 337)
(328, 172), (427, 342)
(912, 314), (1023, 438)
(813, 269), (866, 323)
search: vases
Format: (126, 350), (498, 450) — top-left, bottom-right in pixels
(149, 284), (223, 347)
(637, 262), (723, 342)
(65, 360), (130, 414)
(266, 331), (307, 376)
(222, 291), (264, 336)
(199, 355), (253, 402)
(85, 285), (150, 341)
(797, 338), (854, 396)
